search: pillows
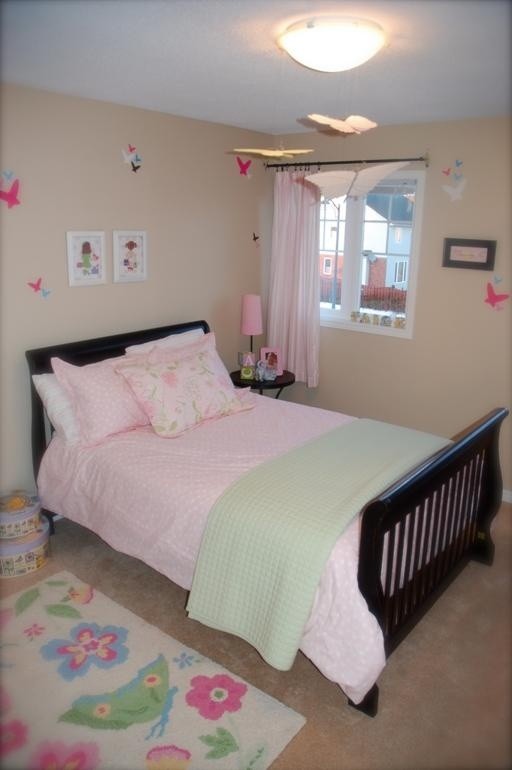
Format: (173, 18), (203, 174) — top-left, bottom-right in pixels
(31, 326), (256, 451)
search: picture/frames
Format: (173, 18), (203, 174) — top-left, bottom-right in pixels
(65, 229), (107, 287)
(112, 229), (149, 285)
(441, 237), (497, 271)
(260, 347), (284, 376)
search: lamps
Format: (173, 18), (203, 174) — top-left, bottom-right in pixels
(272, 12), (388, 74)
(240, 294), (264, 352)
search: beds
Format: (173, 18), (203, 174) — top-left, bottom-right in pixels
(24, 319), (509, 717)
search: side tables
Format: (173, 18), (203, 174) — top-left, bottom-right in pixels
(230, 369), (296, 399)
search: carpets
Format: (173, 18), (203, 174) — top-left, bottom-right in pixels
(0, 560), (307, 770)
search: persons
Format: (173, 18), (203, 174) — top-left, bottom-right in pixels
(267, 353), (276, 364)
